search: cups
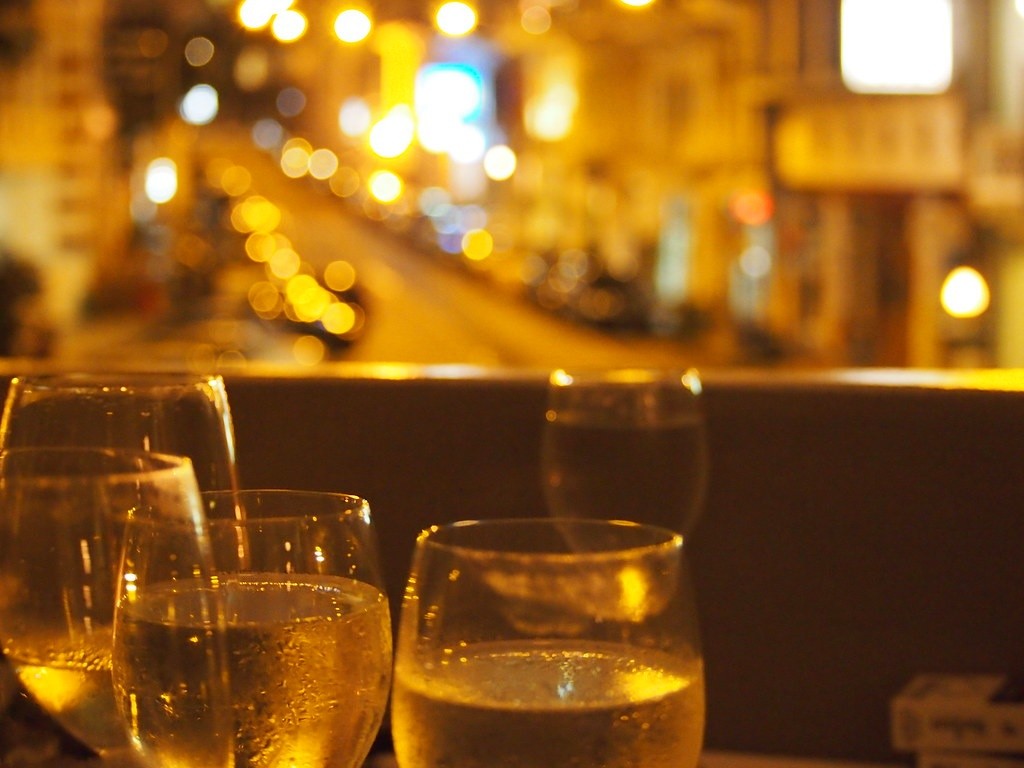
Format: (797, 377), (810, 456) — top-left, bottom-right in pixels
(391, 518), (706, 768)
(0, 452), (231, 768)
(110, 489), (392, 768)
(0, 376), (250, 577)
(537, 369), (709, 640)
